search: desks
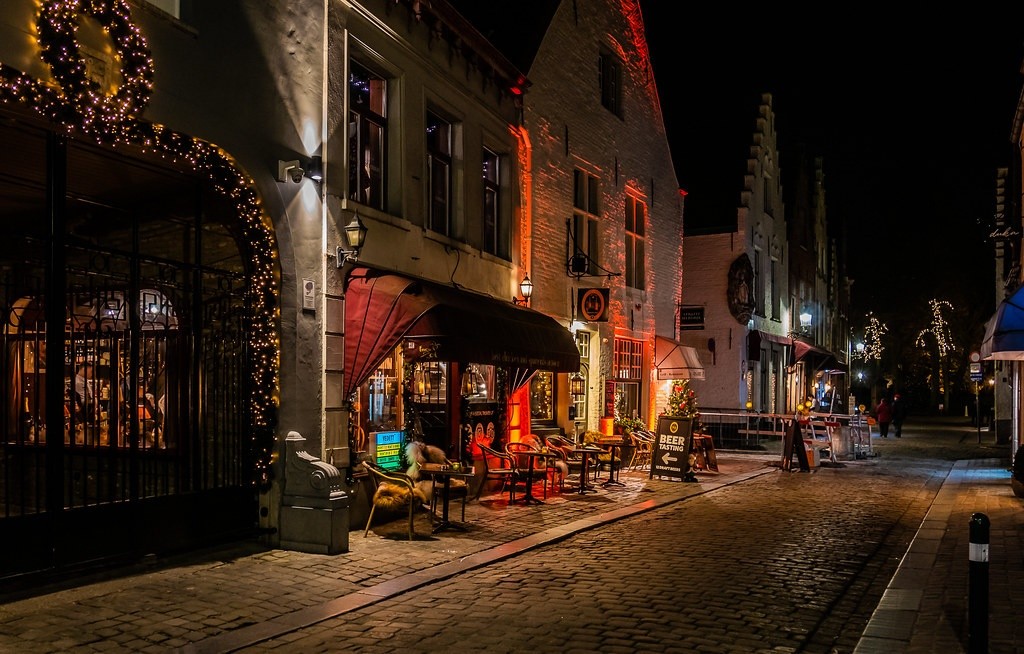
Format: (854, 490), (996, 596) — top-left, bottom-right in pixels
(417, 463), (476, 533)
(509, 450), (556, 506)
(567, 449), (610, 495)
(597, 441), (636, 488)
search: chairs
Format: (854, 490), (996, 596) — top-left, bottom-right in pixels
(476, 431), (655, 504)
(403, 443), (468, 522)
(360, 461), (427, 541)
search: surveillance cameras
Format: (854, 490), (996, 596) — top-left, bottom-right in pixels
(291, 168), (304, 183)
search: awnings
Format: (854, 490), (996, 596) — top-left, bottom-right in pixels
(749, 329), (793, 346)
(343, 265), (580, 407)
(791, 341), (835, 378)
(979, 282), (1024, 361)
(828, 360), (848, 375)
(655, 335), (705, 381)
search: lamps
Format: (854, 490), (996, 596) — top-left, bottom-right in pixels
(511, 272), (532, 303)
(850, 343), (865, 363)
(791, 313), (812, 342)
(308, 154), (323, 180)
(334, 210), (368, 267)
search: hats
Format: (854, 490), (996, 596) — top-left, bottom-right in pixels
(894, 394), (901, 399)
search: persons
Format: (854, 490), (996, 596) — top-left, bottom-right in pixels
(890, 394), (905, 438)
(76, 361), (93, 402)
(808, 394), (820, 421)
(99, 365), (123, 404)
(877, 398), (891, 438)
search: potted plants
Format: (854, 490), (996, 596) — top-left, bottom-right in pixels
(1011, 444), (1024, 499)
